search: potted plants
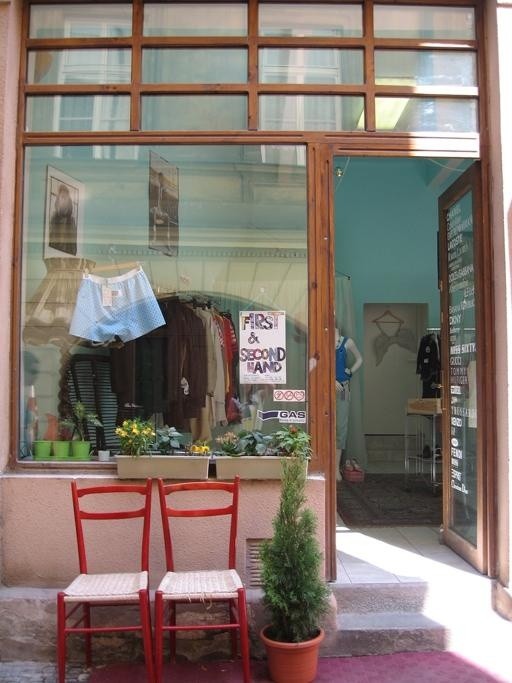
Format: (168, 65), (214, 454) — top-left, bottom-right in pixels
(255, 455), (334, 681)
(30, 401), (102, 460)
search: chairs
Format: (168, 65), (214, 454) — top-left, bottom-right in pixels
(152, 474), (252, 682)
(56, 476), (154, 682)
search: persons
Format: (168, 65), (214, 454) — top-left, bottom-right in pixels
(151, 172), (173, 252)
(336, 329), (363, 481)
(23, 350), (40, 385)
(235, 378), (277, 433)
(49, 185), (76, 256)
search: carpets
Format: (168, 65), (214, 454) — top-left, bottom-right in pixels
(93, 648), (500, 683)
(336, 471), (443, 529)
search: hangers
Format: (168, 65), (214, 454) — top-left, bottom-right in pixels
(422, 327), (441, 346)
(372, 307), (404, 324)
(140, 286), (236, 333)
(77, 249), (140, 276)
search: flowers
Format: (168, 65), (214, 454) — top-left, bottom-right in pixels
(115, 414), (210, 457)
(213, 425), (312, 454)
